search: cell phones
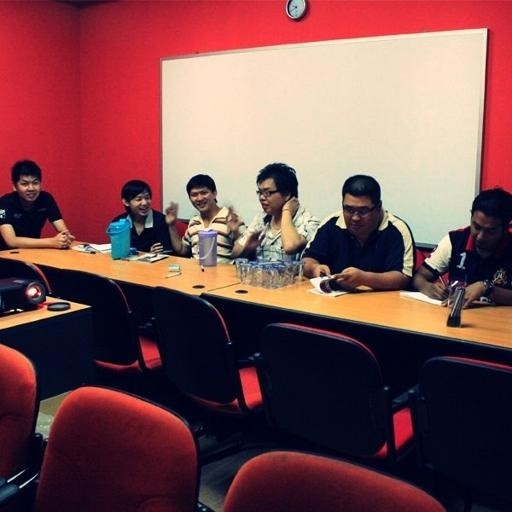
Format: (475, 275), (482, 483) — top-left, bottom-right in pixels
(168, 263), (180, 271)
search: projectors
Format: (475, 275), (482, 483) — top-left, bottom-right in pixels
(0, 277), (46, 315)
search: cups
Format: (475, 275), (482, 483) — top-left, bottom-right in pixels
(107, 219), (135, 259)
(198, 231), (218, 266)
(236, 257), (305, 289)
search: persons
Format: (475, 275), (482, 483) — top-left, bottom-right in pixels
(105, 179), (174, 255)
(300, 174), (415, 292)
(226, 159), (324, 272)
(162, 172), (249, 263)
(410, 187), (512, 310)
(1, 159), (77, 250)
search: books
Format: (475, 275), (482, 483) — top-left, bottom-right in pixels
(401, 287), (461, 307)
(305, 273), (352, 297)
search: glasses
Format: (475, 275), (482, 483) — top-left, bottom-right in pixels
(256, 191), (278, 197)
(342, 205), (378, 214)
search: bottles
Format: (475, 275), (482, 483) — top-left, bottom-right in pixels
(445, 287), (465, 328)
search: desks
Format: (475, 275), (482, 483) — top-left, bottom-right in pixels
(0, 237), (255, 300)
(0, 295), (92, 402)
(200, 266), (512, 358)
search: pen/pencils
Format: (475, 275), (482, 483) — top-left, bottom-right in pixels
(165, 272), (182, 278)
(11, 252), (19, 253)
(201, 263), (204, 272)
(438, 273), (447, 287)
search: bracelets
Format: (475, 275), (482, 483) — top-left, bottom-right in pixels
(280, 209), (294, 217)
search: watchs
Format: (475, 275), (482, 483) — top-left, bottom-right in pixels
(481, 279), (494, 297)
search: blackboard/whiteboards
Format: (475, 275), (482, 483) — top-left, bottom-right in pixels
(160, 28), (489, 249)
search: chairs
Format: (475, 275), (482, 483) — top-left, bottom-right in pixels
(9, 257), (53, 293)
(221, 450), (446, 510)
(70, 275), (162, 399)
(1, 344), (48, 508)
(254, 320), (420, 478)
(3, 385), (206, 511)
(407, 354), (510, 512)
(151, 283), (279, 465)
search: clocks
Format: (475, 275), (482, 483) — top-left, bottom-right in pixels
(285, 0), (308, 20)
